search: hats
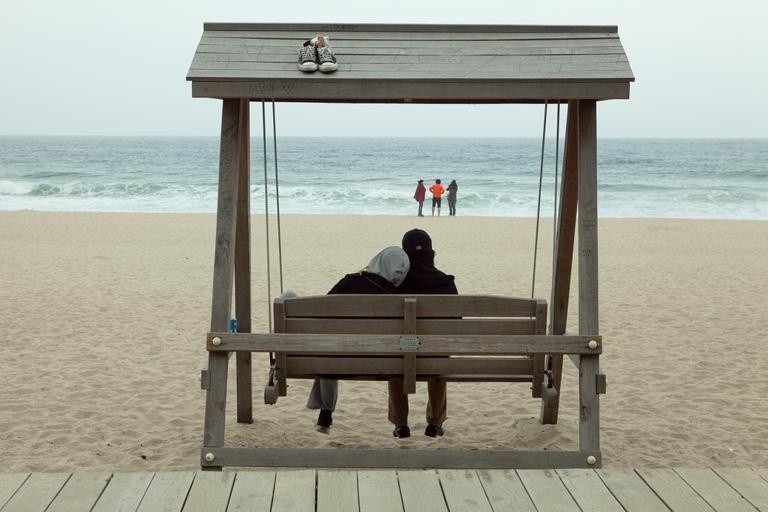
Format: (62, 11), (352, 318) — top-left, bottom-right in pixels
(402, 228), (432, 253)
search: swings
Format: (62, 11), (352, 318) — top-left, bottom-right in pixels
(262, 99), (561, 406)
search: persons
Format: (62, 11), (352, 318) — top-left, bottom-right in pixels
(412, 179), (427, 217)
(428, 179), (445, 217)
(305, 245), (412, 435)
(384, 227), (463, 439)
(445, 179), (458, 216)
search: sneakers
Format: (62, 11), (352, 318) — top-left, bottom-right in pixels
(393, 426), (410, 438)
(424, 424), (444, 438)
(313, 409), (333, 434)
